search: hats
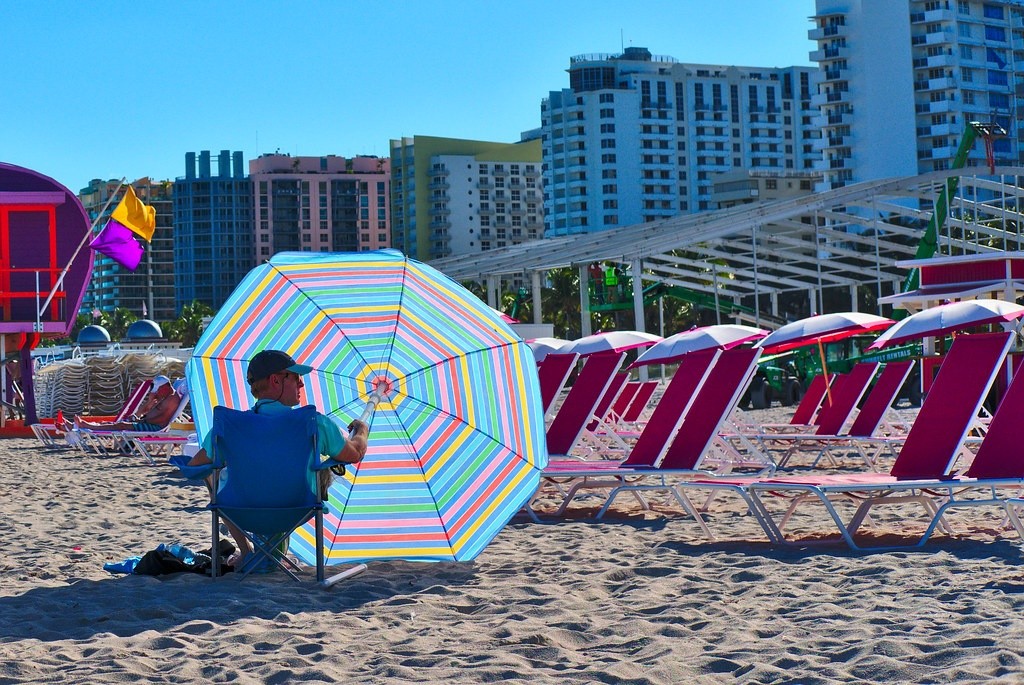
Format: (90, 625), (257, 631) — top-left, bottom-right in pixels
(247, 350), (313, 385)
(151, 375), (170, 393)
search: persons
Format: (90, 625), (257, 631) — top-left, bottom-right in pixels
(189, 350), (367, 574)
(54, 375), (181, 432)
(7, 359), (24, 419)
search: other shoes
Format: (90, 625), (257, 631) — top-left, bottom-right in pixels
(228, 550), (253, 565)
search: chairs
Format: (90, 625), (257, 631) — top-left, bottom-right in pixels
(31, 332), (1024, 591)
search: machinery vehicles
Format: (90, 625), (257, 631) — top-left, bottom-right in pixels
(511, 282), (532, 320)
(587, 103), (1017, 411)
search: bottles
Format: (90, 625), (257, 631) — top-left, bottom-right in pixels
(170, 546), (196, 564)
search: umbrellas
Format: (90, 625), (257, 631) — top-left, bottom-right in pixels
(489, 299), (1024, 406)
(184, 248), (549, 563)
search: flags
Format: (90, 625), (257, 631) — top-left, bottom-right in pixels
(88, 185), (158, 271)
